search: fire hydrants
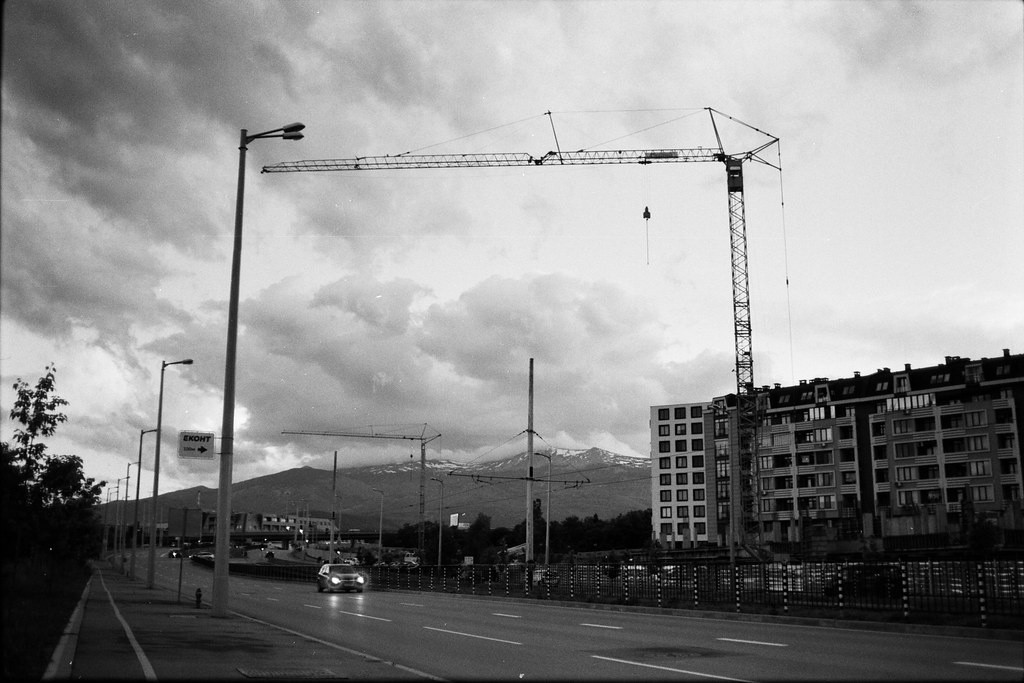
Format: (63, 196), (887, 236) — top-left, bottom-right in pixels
(196, 587), (202, 604)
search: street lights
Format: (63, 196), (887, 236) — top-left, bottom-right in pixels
(373, 488), (384, 565)
(213, 122), (307, 617)
(149, 359), (194, 586)
(534, 452), (551, 566)
(104, 427), (159, 582)
(431, 477), (442, 566)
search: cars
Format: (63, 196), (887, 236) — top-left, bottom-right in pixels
(188, 552), (214, 559)
(168, 548), (183, 558)
(534, 570), (559, 585)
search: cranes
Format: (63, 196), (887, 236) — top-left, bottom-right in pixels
(282, 422), (441, 566)
(262, 107), (782, 566)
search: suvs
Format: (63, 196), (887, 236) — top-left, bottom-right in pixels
(317, 564), (366, 594)
